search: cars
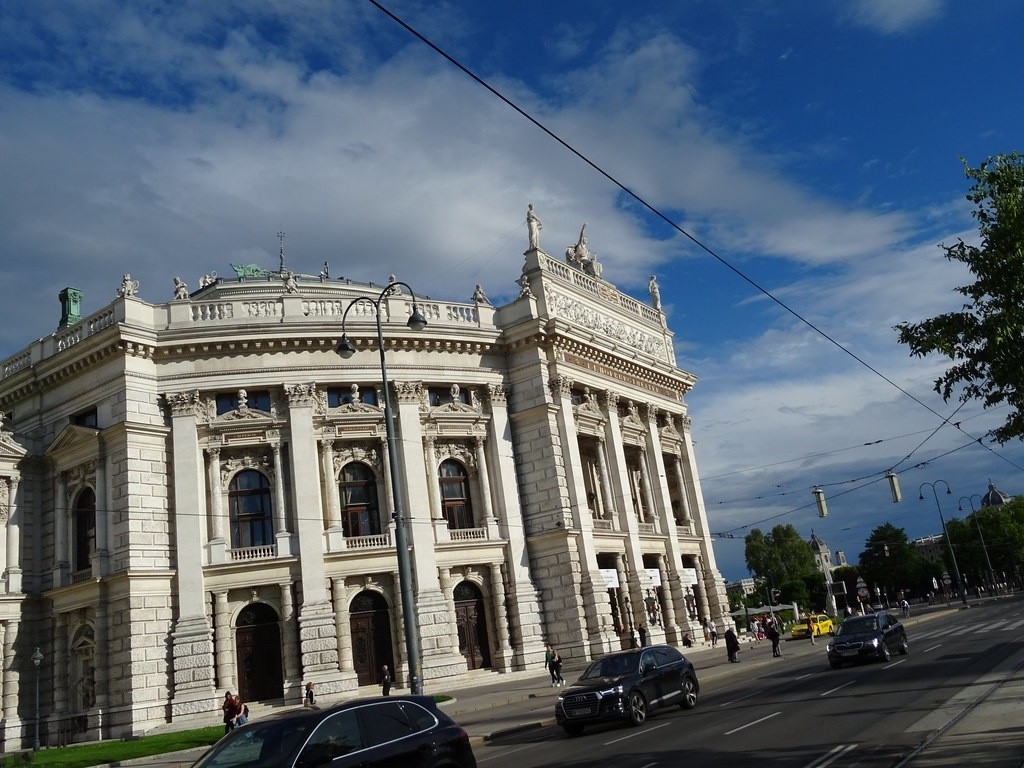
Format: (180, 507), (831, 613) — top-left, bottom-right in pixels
(792, 613), (833, 640)
(826, 612), (909, 669)
(556, 645), (700, 735)
(189, 689), (477, 768)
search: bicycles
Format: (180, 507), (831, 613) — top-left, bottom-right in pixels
(903, 607), (910, 618)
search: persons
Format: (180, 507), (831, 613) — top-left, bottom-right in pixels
(526, 204), (543, 248)
(382, 665), (392, 696)
(750, 613), (786, 657)
(473, 285), (486, 305)
(449, 384), (460, 401)
(172, 277), (189, 300)
(73, 667), (95, 709)
(305, 682), (316, 705)
(638, 623), (647, 647)
(350, 384), (360, 402)
(844, 604), (874, 618)
(901, 598), (910, 618)
(703, 618), (742, 663)
(648, 275), (662, 310)
(807, 613), (818, 645)
(986, 582), (993, 596)
(280, 271), (299, 294)
(388, 274), (400, 295)
(237, 389), (249, 407)
(683, 633), (693, 648)
(974, 585), (982, 598)
(545, 644), (566, 686)
(519, 275), (532, 297)
(121, 273), (134, 296)
(222, 691), (248, 735)
(929, 591), (935, 605)
(575, 221), (602, 279)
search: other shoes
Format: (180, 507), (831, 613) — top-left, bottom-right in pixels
(556, 683), (560, 687)
(551, 683), (555, 686)
(562, 680), (565, 685)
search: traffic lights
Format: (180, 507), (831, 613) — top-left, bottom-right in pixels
(771, 588), (781, 606)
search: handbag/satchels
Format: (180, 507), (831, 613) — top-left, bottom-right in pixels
(305, 698), (317, 706)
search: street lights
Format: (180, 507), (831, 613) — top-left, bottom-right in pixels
(335, 283), (427, 694)
(919, 480), (967, 605)
(32, 647), (42, 752)
(958, 493), (1000, 595)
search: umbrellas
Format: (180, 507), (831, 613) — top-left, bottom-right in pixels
(732, 604), (793, 618)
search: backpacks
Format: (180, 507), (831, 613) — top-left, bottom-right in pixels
(241, 704), (249, 718)
